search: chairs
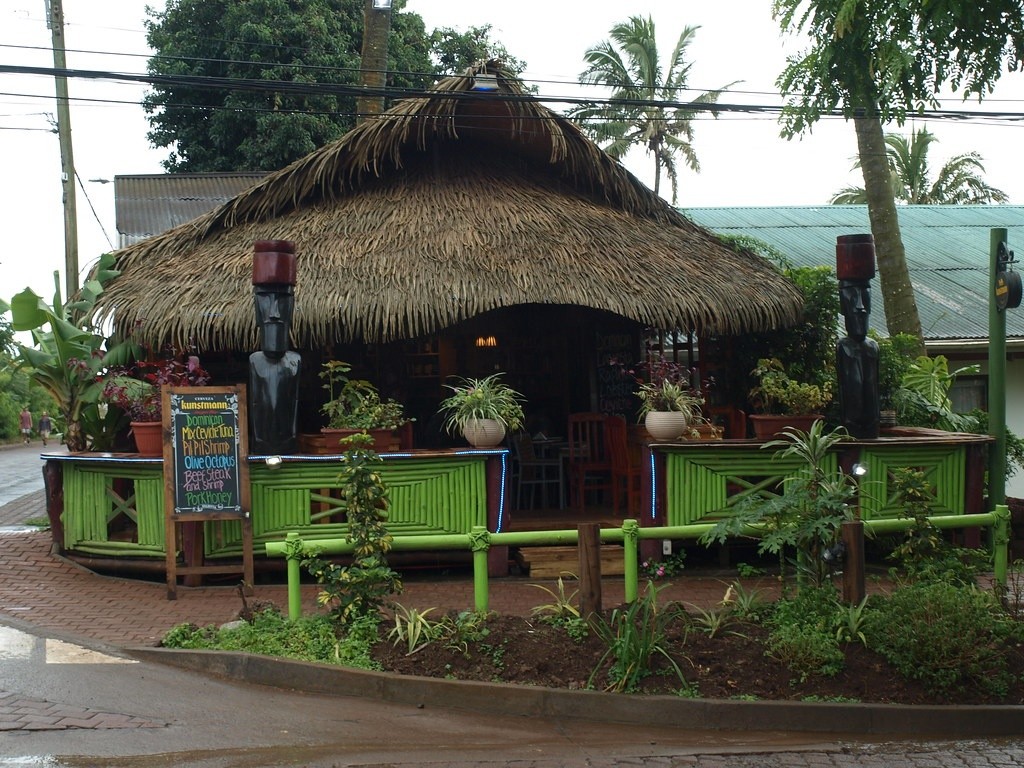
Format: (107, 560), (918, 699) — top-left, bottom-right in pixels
(604, 415), (641, 518)
(567, 412), (610, 511)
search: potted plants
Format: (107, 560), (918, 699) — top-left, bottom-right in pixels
(321, 382), (400, 455)
(67, 315), (211, 458)
(747, 357), (825, 441)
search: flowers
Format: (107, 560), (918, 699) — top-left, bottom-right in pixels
(441, 373), (524, 430)
(633, 379), (722, 440)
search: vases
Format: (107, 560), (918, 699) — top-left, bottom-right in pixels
(463, 419), (504, 448)
(644, 411), (686, 440)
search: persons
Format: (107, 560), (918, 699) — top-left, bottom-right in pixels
(835, 279), (881, 436)
(37, 411), (51, 448)
(20, 408), (32, 448)
(248, 285), (302, 451)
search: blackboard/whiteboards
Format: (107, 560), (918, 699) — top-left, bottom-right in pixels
(161, 384), (252, 521)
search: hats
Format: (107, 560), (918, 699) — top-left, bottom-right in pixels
(41, 412), (47, 416)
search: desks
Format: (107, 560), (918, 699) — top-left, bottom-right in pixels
(533, 442), (579, 510)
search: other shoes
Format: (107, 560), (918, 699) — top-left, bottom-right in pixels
(44, 443), (47, 448)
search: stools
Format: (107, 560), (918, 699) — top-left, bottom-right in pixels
(514, 434), (560, 517)
